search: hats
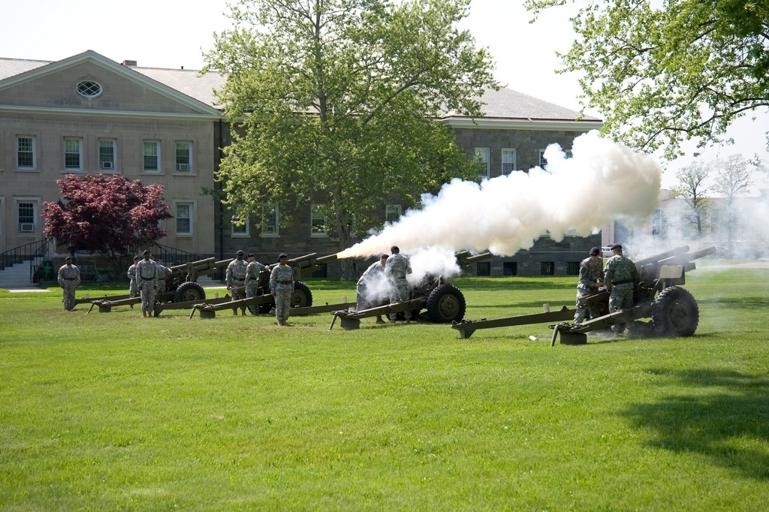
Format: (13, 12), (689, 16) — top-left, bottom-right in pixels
(590, 248), (599, 256)
(611, 245), (621, 250)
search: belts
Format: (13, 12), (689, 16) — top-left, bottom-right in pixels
(612, 279), (632, 285)
(232, 276), (244, 281)
(140, 275), (153, 280)
(64, 278), (75, 280)
(276, 280), (288, 284)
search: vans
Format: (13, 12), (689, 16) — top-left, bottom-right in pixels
(601, 247), (626, 257)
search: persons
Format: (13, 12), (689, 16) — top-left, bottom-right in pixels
(57, 255), (81, 311)
(135, 250), (159, 316)
(384, 247), (414, 322)
(225, 248), (248, 316)
(129, 256), (140, 299)
(269, 253), (295, 326)
(244, 253), (273, 315)
(356, 254), (392, 323)
(605, 244), (640, 332)
(572, 248), (605, 330)
(158, 261), (172, 296)
